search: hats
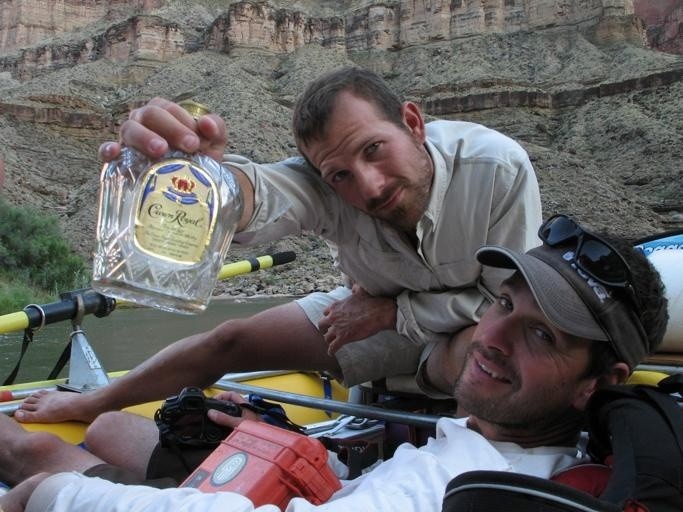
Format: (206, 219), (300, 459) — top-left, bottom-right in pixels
(473, 239), (652, 373)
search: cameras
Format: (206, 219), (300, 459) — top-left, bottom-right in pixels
(154, 385), (241, 448)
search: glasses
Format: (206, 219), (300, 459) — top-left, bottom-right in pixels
(538, 214), (642, 305)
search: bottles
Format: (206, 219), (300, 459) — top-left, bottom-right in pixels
(92, 102), (244, 317)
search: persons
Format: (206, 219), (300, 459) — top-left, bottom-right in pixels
(0, 214), (670, 512)
(13, 68), (546, 423)
